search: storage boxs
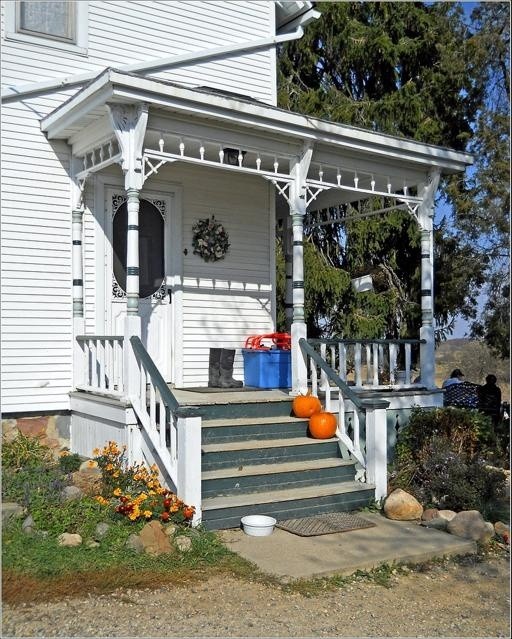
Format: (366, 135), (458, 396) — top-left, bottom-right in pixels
(240, 347), (295, 390)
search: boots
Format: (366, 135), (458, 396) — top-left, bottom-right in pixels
(217, 349), (243, 388)
(208, 348), (224, 387)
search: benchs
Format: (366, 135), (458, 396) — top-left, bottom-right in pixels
(441, 382), (505, 425)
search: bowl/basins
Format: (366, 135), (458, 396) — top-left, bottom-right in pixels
(240, 514), (277, 537)
(349, 272), (374, 295)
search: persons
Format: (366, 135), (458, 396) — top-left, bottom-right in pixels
(482, 374), (501, 424)
(442, 369), (464, 402)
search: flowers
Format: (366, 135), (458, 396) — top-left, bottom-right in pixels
(191, 213), (231, 262)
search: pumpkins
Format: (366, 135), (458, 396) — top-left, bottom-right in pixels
(310, 410), (337, 438)
(291, 391), (320, 417)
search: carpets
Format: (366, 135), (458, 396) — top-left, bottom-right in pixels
(173, 385), (272, 395)
(276, 510), (380, 538)
